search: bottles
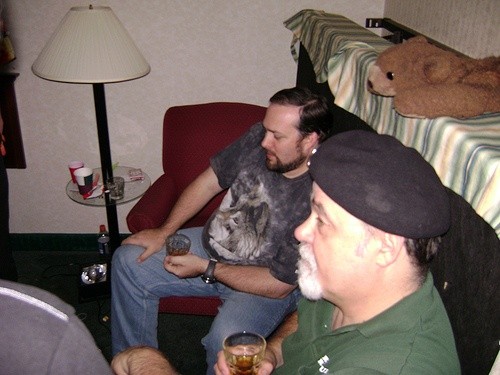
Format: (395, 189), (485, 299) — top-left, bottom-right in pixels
(98, 224), (111, 256)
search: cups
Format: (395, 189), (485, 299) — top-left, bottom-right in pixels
(68, 160), (85, 183)
(165, 234), (191, 266)
(109, 176), (125, 200)
(74, 167), (93, 195)
(222, 330), (266, 375)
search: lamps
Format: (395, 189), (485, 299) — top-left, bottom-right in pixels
(31, 4), (150, 291)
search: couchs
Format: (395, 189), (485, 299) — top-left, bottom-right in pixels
(125, 101), (267, 318)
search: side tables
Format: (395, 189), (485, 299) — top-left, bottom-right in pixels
(66, 165), (152, 206)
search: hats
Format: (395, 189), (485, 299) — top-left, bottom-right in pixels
(309, 130), (451, 239)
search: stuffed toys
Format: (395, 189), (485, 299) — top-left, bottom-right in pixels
(368, 36), (500, 118)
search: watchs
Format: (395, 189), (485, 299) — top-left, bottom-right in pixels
(201, 258), (218, 284)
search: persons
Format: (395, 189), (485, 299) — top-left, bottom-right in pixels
(111, 132), (462, 375)
(112, 86), (331, 375)
